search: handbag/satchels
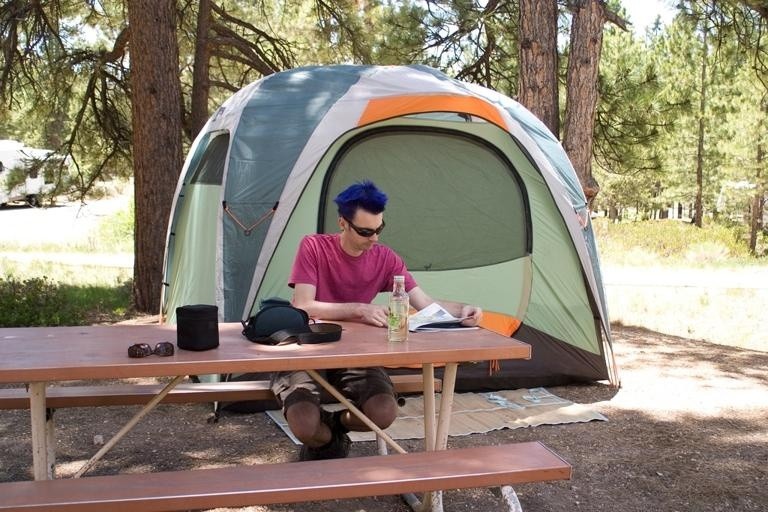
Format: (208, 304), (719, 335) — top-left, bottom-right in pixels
(243, 304), (311, 345)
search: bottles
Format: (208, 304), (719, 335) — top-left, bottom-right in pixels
(388, 276), (408, 343)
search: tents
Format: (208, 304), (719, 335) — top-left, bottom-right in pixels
(159, 63), (622, 389)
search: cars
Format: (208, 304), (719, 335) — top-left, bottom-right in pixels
(0, 140), (73, 208)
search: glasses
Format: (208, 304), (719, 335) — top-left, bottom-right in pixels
(342, 214), (386, 237)
(128, 342), (174, 359)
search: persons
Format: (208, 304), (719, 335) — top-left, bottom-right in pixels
(267, 180), (484, 459)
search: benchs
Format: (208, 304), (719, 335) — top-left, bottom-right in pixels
(2, 373), (443, 413)
(0, 436), (572, 511)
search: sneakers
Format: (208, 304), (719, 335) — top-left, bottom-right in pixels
(299, 408), (351, 460)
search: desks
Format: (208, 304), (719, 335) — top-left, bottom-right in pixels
(0, 318), (535, 510)
